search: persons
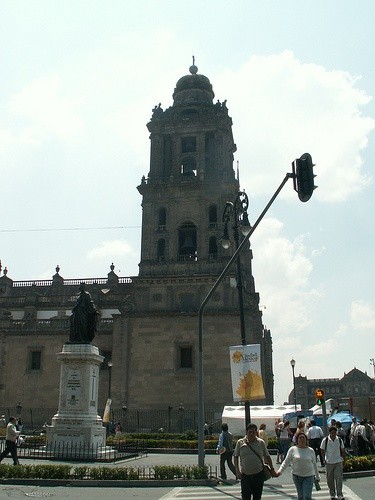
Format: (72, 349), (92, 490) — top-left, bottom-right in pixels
(275, 421), (293, 464)
(319, 427), (346, 500)
(115, 422), (122, 437)
(275, 419), (285, 438)
(217, 422), (240, 481)
(335, 421), (346, 445)
(0, 416), (24, 465)
(67, 283), (99, 343)
(204, 421), (209, 435)
(258, 423), (269, 447)
(291, 421), (307, 445)
(209, 422), (215, 435)
(306, 419), (326, 467)
(330, 419), (337, 429)
(276, 432), (321, 500)
(349, 416), (375, 456)
(234, 423), (277, 500)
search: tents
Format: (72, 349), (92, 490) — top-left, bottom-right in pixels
(221, 404), (302, 438)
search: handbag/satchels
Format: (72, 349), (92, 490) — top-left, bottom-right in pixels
(219, 447), (226, 455)
(264, 465), (271, 481)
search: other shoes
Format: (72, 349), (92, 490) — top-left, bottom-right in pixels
(331, 496), (344, 500)
(276, 461), (280, 464)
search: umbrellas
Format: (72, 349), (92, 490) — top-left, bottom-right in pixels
(325, 412), (363, 427)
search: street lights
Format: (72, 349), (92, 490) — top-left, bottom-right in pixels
(221, 190), (253, 432)
(290, 357), (297, 411)
(107, 359), (113, 398)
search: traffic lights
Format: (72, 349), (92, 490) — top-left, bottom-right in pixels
(296, 152), (318, 203)
(315, 388), (326, 406)
